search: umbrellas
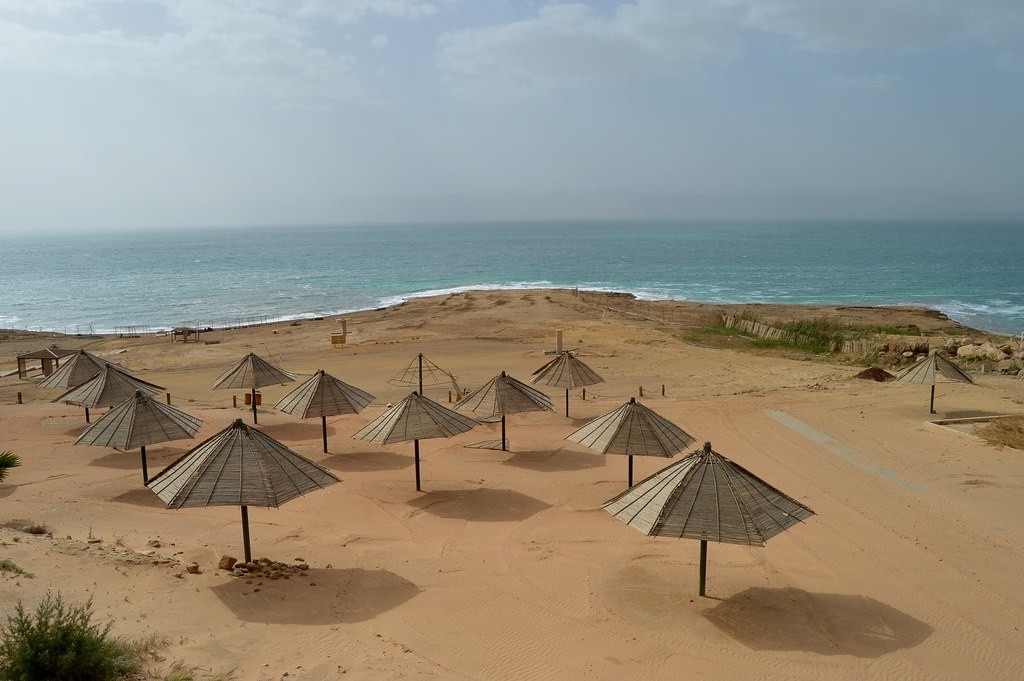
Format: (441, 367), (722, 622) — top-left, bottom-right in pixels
(35, 343), (817, 601)
(894, 352), (977, 415)
